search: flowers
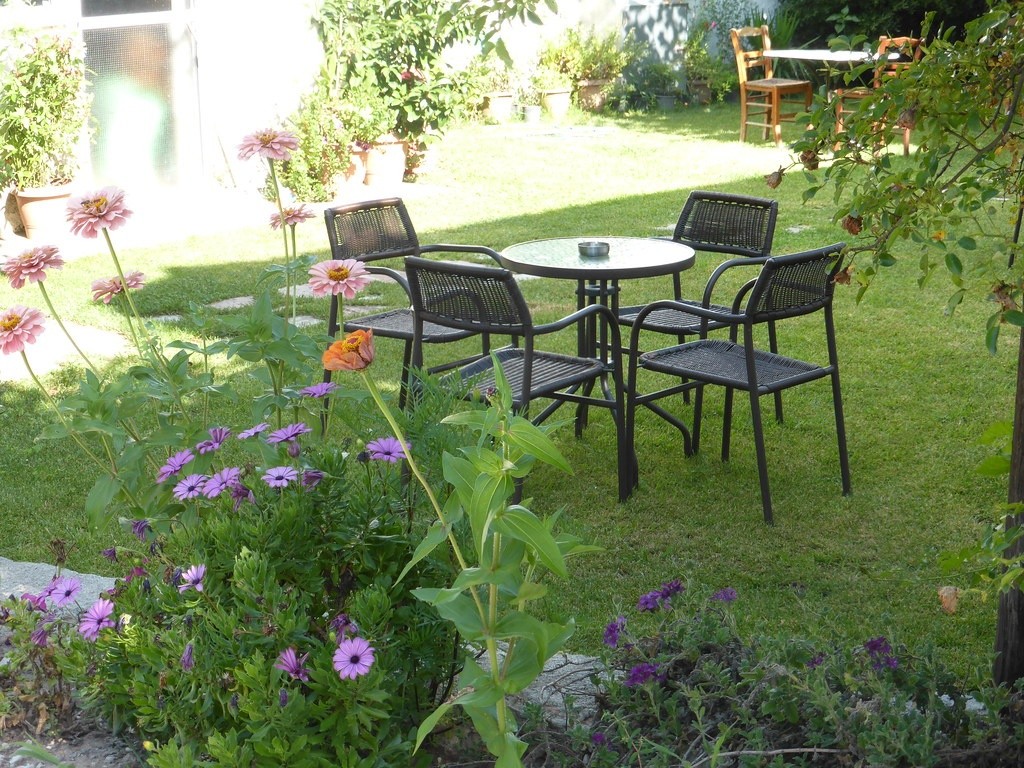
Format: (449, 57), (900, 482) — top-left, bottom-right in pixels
(0, 129), (988, 768)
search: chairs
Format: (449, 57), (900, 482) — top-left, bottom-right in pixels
(833, 36), (925, 158)
(730, 25), (814, 147)
(599, 191), (785, 452)
(396, 256), (628, 521)
(626, 243), (851, 524)
(321, 197), (520, 442)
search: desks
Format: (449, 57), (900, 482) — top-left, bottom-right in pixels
(763, 50), (900, 145)
(498, 237), (697, 489)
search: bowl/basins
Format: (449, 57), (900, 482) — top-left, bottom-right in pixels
(578, 242), (610, 256)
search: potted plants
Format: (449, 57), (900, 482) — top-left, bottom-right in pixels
(0, 0), (738, 240)
